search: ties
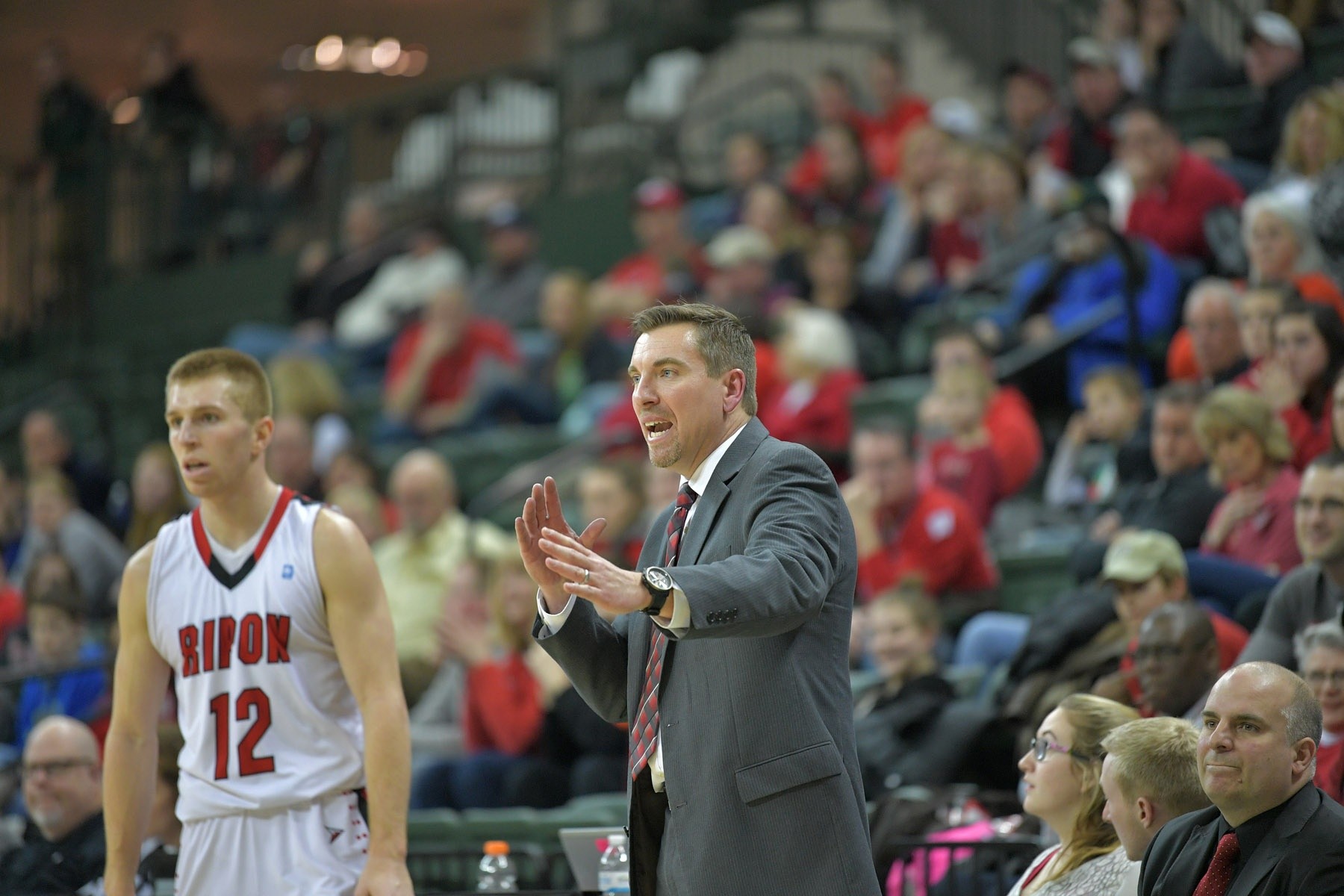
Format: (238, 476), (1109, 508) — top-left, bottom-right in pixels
(1192, 832), (1241, 896)
(628, 482), (697, 780)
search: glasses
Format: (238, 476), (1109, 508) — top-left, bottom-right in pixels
(1030, 736), (1093, 763)
(15, 757), (91, 782)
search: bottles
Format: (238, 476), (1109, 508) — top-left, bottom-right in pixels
(598, 834), (631, 896)
(478, 840), (518, 895)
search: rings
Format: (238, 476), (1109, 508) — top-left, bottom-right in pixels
(579, 570), (590, 585)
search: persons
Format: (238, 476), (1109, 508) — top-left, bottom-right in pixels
(101, 347), (413, 896)
(514, 304), (881, 896)
(1, 14), (1343, 895)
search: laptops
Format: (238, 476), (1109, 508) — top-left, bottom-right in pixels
(559, 826), (628, 891)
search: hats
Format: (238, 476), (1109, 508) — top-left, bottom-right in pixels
(1102, 528), (1188, 585)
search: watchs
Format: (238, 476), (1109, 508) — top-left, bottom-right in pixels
(641, 566), (673, 616)
